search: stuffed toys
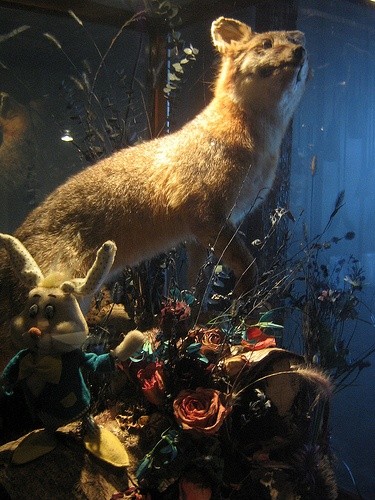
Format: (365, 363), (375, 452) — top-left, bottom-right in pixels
(0, 232), (144, 467)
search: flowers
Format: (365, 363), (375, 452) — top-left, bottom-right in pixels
(87, 155), (375, 500)
